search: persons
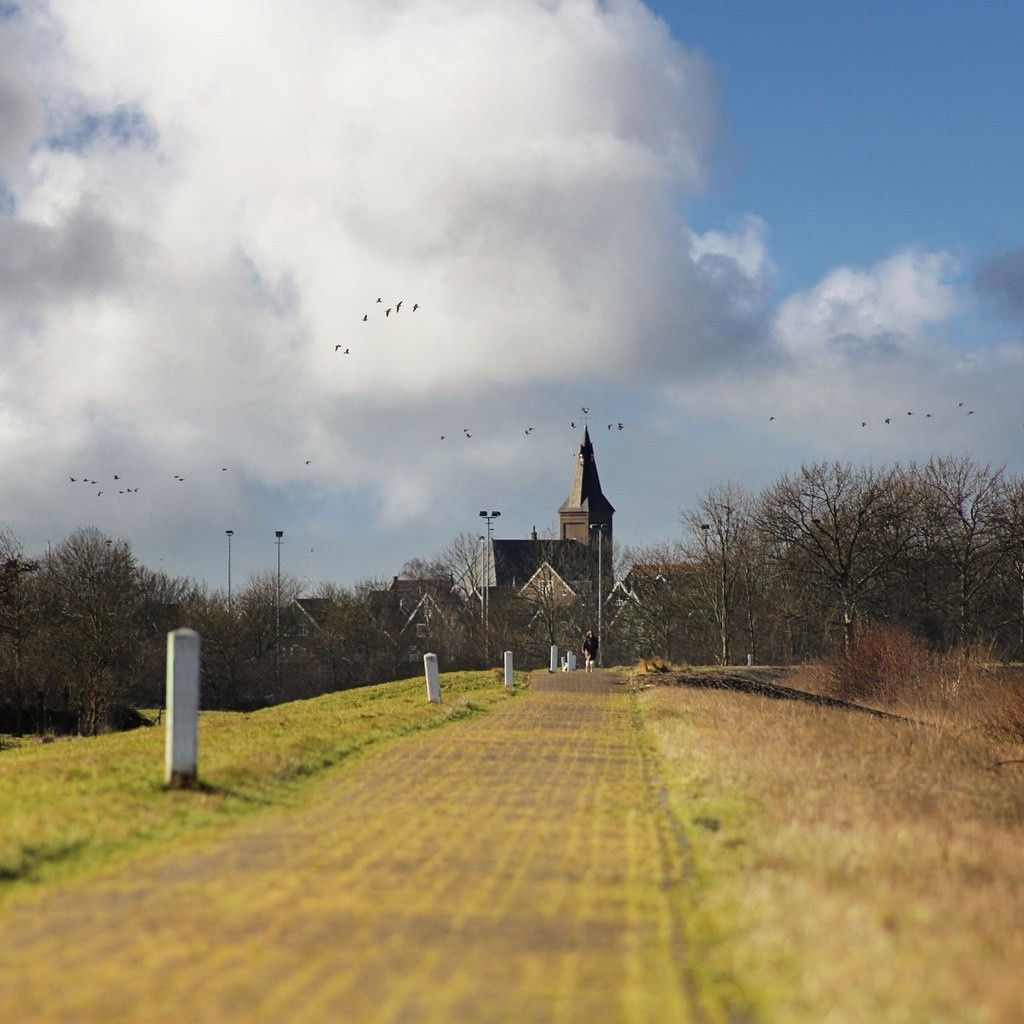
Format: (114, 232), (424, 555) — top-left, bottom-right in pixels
(582, 631), (599, 673)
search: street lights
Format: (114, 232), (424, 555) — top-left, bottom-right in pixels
(589, 523), (608, 669)
(478, 510), (503, 665)
(273, 528), (283, 698)
(225, 528), (235, 606)
(700, 523), (711, 549)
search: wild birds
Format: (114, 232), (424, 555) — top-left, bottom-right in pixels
(330, 292), (431, 354)
(65, 463), (230, 499)
(433, 412), (651, 439)
(305, 460), (311, 465)
(767, 402), (979, 429)
